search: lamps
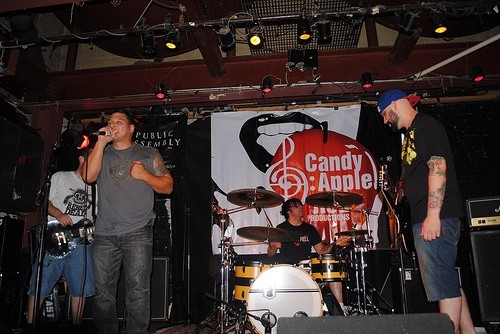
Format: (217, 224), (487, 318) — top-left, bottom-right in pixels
(362, 72), (373, 89)
(398, 10), (449, 36)
(139, 29), (179, 53)
(216, 19), (265, 52)
(469, 65), (484, 82)
(297, 16), (332, 42)
(261, 75), (273, 93)
(155, 83), (170, 100)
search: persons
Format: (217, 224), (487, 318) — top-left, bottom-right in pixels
(26, 145), (98, 326)
(268, 198), (348, 317)
(79, 110), (174, 334)
(376, 88), (478, 334)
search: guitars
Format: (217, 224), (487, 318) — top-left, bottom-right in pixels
(43, 218), (94, 260)
(380, 165), (415, 251)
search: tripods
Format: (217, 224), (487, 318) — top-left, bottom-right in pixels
(192, 201), (259, 334)
(333, 202), (395, 315)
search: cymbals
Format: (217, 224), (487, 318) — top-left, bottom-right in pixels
(335, 229), (373, 235)
(236, 226), (299, 242)
(305, 191), (362, 207)
(227, 188), (284, 208)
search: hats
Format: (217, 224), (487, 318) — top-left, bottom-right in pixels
(377, 89), (407, 113)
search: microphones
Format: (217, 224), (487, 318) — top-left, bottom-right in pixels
(90, 131), (106, 136)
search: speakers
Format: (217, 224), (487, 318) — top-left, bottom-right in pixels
(399, 267), (462, 314)
(0, 118), (43, 212)
(0, 217), (25, 334)
(470, 231), (500, 325)
(66, 258), (172, 322)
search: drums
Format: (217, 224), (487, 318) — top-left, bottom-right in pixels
(310, 254), (350, 282)
(233, 262), (291, 300)
(246, 264), (324, 334)
